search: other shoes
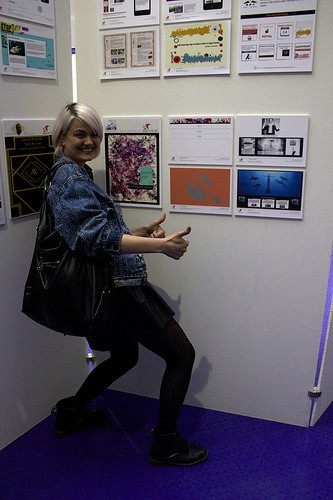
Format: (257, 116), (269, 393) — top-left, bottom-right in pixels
(148, 427), (209, 465)
(53, 395), (107, 438)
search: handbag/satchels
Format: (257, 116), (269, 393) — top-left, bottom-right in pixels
(22, 162), (116, 337)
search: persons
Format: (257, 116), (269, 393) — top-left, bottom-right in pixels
(262, 117), (280, 135)
(43, 102), (211, 467)
